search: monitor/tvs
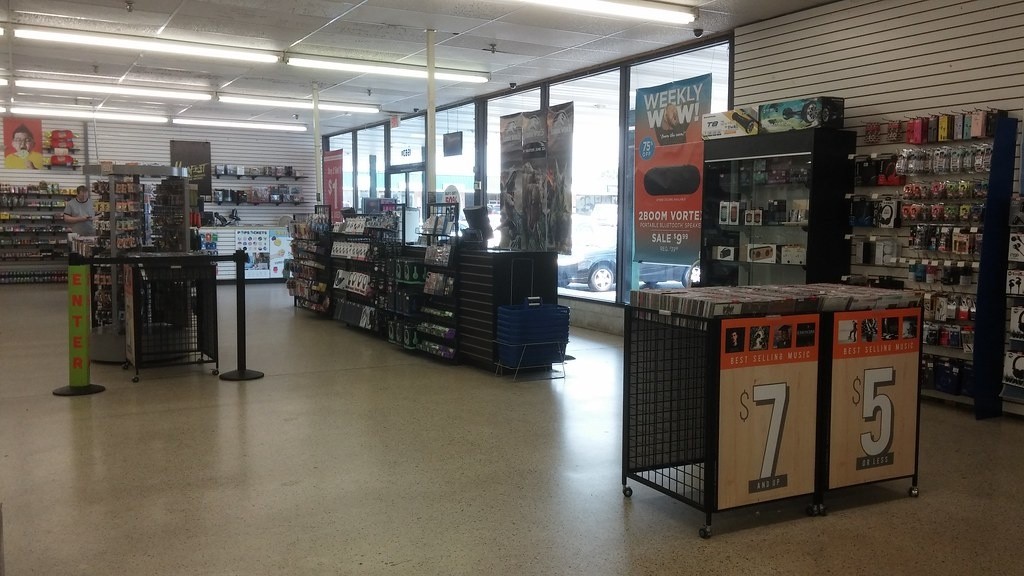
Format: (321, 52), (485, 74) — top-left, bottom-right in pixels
(463, 206), (494, 240)
(339, 207), (357, 219)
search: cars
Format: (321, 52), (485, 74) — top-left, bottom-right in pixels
(488, 200), (500, 207)
(487, 204), (493, 209)
(557, 203), (700, 292)
(487, 206), (493, 214)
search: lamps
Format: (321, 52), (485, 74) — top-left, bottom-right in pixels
(0, 75), (383, 133)
(512, 0), (699, 25)
(0, 20), (491, 86)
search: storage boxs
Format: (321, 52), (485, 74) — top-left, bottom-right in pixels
(376, 256), (430, 351)
(330, 242), (375, 263)
(334, 218), (378, 237)
(0, 213), (54, 220)
(863, 108), (1007, 143)
(849, 156), (906, 230)
(1001, 195), (1024, 391)
(215, 164), (304, 176)
(214, 185), (305, 203)
(334, 299), (379, 332)
(701, 96), (846, 140)
(334, 268), (378, 298)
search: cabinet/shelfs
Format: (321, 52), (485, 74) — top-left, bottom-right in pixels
(284, 205), (332, 314)
(699, 132), (856, 286)
(0, 192), (76, 283)
(1000, 193), (1024, 428)
(212, 173), (311, 206)
(846, 105), (1018, 420)
(333, 203), (406, 336)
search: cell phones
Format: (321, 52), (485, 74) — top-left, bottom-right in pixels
(731, 207), (737, 222)
(721, 206), (727, 222)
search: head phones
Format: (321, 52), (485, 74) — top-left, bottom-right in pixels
(1018, 310), (1024, 333)
(1012, 356), (1024, 379)
(879, 205), (893, 225)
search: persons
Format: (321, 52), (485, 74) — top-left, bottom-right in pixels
(728, 331), (743, 352)
(848, 320), (857, 342)
(6, 125), (42, 169)
(778, 330), (791, 348)
(907, 323), (916, 337)
(64, 186), (96, 236)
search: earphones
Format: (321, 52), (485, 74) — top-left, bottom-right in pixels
(1011, 235), (1022, 242)
(1016, 277), (1021, 286)
(1013, 216), (1023, 225)
(1009, 278), (1014, 288)
(1013, 243), (1021, 251)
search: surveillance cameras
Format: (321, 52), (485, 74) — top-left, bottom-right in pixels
(414, 109), (419, 113)
(694, 29), (703, 37)
(510, 83), (517, 89)
